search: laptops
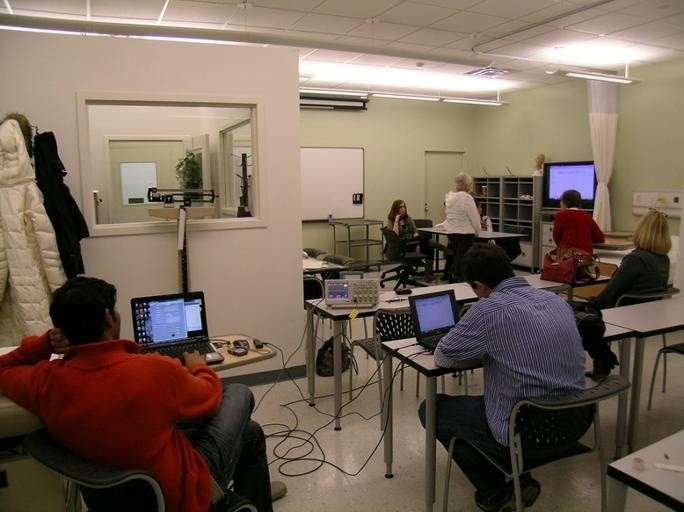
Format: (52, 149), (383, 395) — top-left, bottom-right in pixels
(407, 289), (460, 354)
(130, 290), (225, 365)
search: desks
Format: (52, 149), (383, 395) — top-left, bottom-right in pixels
(379, 320), (635, 511)
(305, 272), (612, 430)
(606, 429), (683, 511)
(0, 332), (288, 505)
(416, 226), (528, 283)
(599, 293), (684, 458)
(303, 257), (348, 275)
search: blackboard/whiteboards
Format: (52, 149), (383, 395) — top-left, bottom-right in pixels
(298, 146), (365, 223)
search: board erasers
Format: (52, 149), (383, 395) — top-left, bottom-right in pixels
(352, 193), (363, 204)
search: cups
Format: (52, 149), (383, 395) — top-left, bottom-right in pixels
(482, 186), (487, 197)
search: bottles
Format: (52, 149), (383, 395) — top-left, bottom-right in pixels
(329, 212), (333, 222)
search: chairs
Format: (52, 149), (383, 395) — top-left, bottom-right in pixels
(349, 307), (417, 429)
(442, 373), (632, 510)
(614, 286), (674, 394)
(648, 342), (684, 409)
(377, 219), (435, 290)
(302, 275), (353, 337)
(20, 430), (258, 511)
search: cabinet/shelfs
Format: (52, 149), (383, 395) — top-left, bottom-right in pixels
(472, 174), (542, 271)
(331, 217), (385, 266)
(535, 207), (560, 273)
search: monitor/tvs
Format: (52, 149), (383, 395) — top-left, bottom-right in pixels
(541, 160), (597, 213)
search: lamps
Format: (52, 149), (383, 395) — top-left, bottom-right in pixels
(441, 86), (510, 107)
(545, 45), (641, 85)
(299, 86), (371, 101)
(368, 90), (442, 102)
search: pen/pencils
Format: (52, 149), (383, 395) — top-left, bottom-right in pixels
(386, 299), (407, 302)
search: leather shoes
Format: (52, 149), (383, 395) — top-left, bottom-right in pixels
(584, 350), (619, 382)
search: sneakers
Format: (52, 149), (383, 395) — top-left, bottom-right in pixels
(474, 472), (541, 512)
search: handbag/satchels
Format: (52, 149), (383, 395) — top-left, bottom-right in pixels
(540, 247), (598, 284)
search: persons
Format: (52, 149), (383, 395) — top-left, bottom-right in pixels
(552, 189), (605, 257)
(475, 201), (493, 232)
(571, 206), (673, 382)
(417, 240), (588, 512)
(440, 172), (481, 282)
(386, 198), (447, 283)
(1, 274), (287, 511)
